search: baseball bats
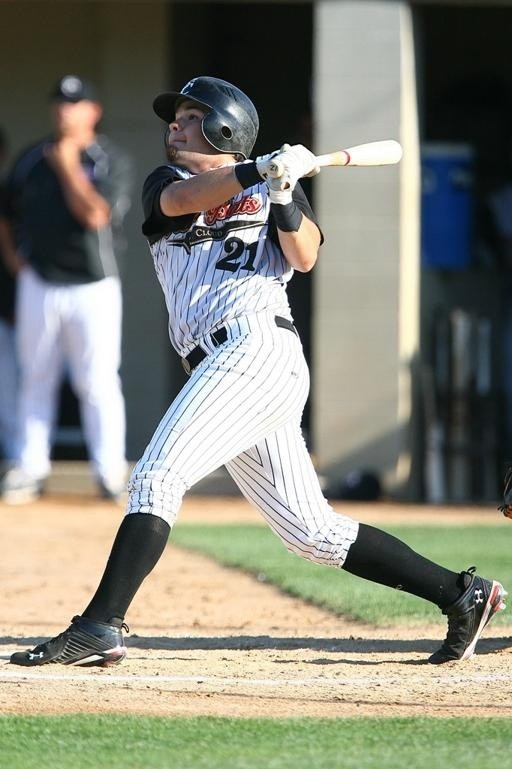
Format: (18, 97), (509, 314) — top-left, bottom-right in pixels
(267, 139), (403, 178)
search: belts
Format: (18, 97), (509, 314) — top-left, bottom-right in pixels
(180, 313), (298, 380)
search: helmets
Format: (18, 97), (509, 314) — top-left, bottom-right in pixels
(152, 73), (263, 161)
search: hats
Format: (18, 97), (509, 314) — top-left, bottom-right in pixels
(49, 73), (102, 105)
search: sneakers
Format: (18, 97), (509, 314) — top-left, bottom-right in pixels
(9, 615), (131, 668)
(1, 467), (130, 501)
(427, 564), (508, 669)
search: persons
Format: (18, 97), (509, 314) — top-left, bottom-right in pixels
(1, 74), (137, 503)
(9, 76), (508, 668)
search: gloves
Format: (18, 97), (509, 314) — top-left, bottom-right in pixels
(268, 143), (317, 206)
(253, 143), (323, 183)
